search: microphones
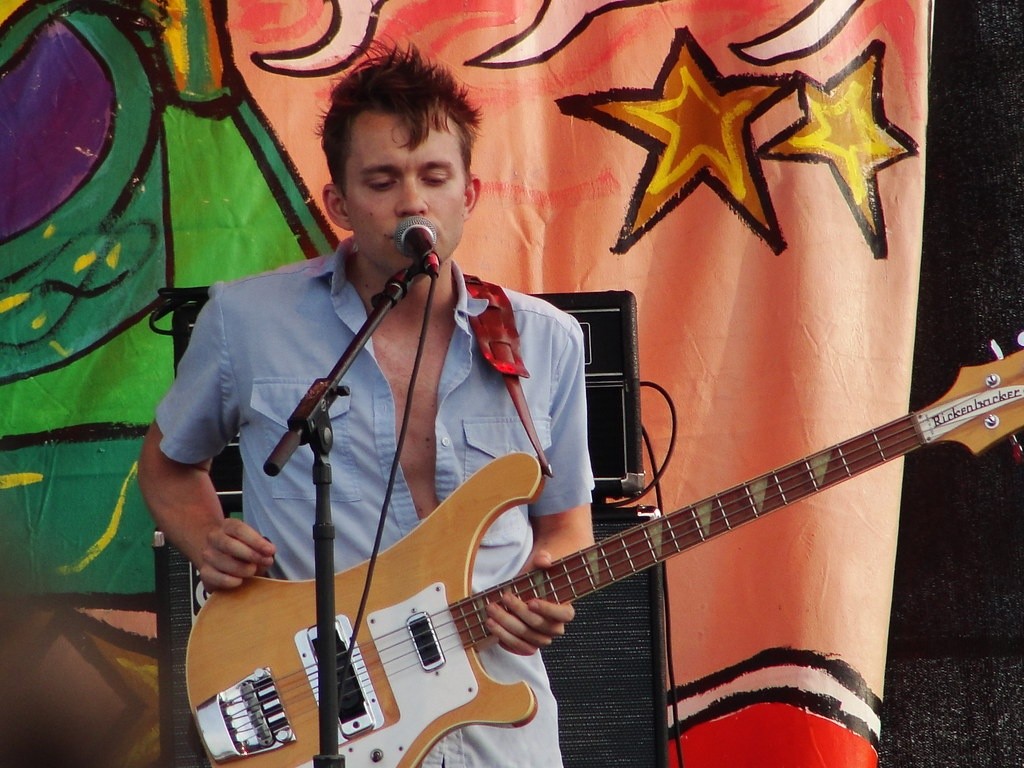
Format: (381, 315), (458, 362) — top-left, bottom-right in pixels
(395, 216), (436, 277)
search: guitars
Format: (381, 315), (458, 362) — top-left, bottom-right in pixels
(184, 334), (1024, 768)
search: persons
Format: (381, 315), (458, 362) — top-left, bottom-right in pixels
(136, 42), (597, 768)
